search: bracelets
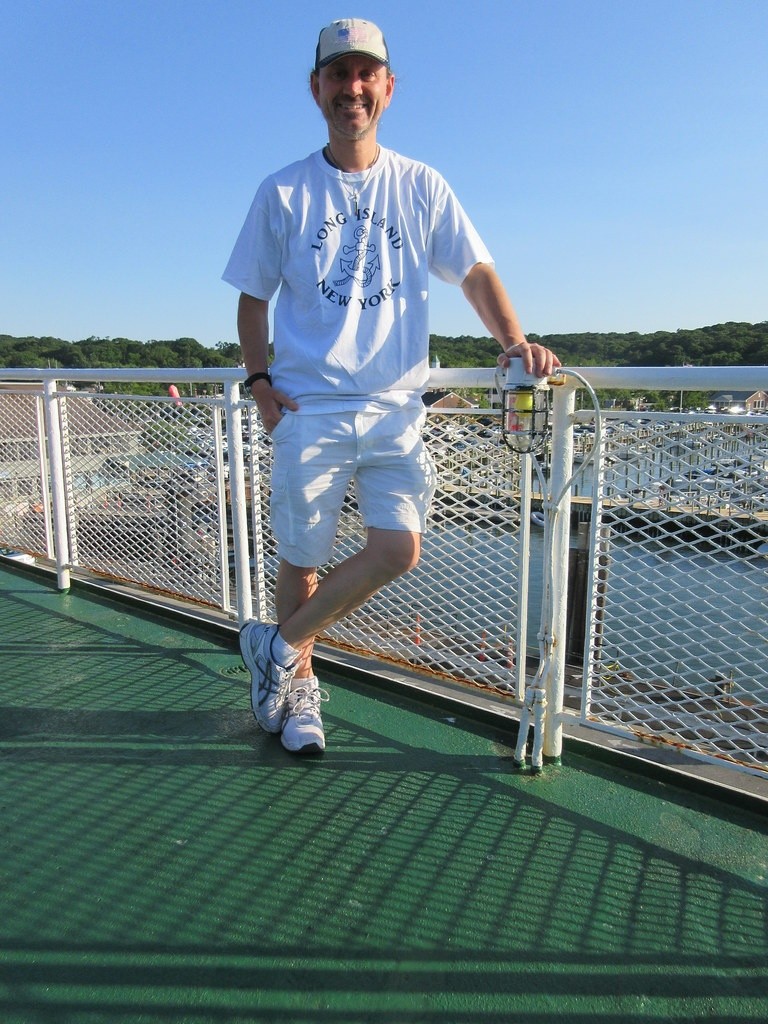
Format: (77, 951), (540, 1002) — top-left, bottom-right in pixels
(244, 373), (271, 388)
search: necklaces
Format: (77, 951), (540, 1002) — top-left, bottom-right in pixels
(325, 139), (378, 214)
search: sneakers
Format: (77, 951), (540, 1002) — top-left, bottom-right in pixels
(238, 619), (295, 734)
(280, 675), (325, 755)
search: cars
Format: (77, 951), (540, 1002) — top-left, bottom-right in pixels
(187, 425), (272, 481)
(573, 405), (768, 436)
(423, 403), (574, 447)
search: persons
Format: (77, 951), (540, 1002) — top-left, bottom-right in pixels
(223, 18), (561, 753)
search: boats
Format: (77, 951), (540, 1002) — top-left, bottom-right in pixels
(624, 425), (768, 509)
(451, 449), (643, 496)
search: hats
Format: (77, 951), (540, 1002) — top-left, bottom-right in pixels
(313, 18), (390, 76)
